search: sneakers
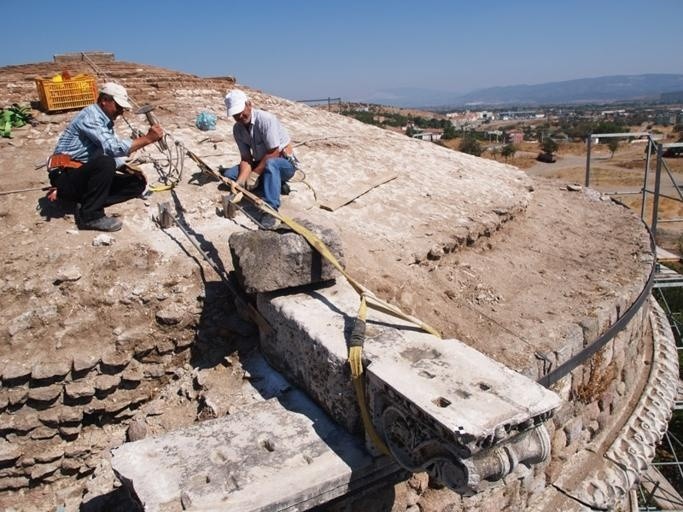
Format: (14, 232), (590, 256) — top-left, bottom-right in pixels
(80, 215), (123, 232)
(262, 210), (281, 230)
(282, 182), (291, 195)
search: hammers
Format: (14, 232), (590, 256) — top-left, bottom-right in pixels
(134, 104), (168, 150)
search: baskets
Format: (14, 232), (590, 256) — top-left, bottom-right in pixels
(33, 72), (99, 111)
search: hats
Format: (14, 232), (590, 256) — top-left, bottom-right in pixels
(99, 82), (133, 108)
(225, 89), (250, 116)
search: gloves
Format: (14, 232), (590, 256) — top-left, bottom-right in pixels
(232, 181), (246, 194)
(247, 172), (259, 190)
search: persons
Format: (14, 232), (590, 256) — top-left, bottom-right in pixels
(44, 81), (163, 233)
(223, 90), (298, 228)
